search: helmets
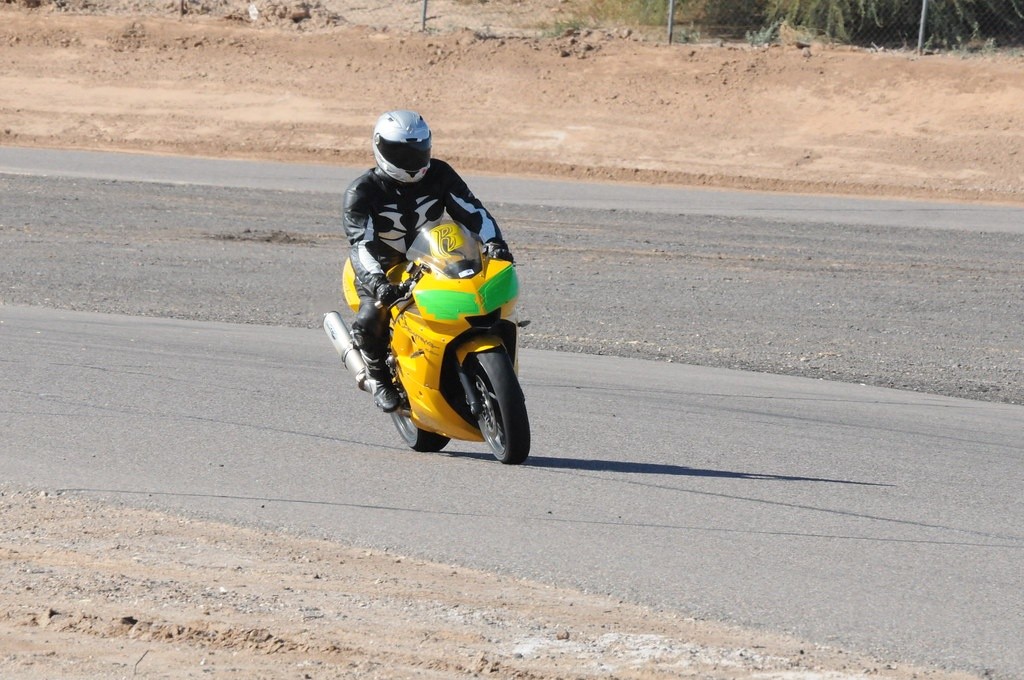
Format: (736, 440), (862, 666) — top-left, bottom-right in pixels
(372, 110), (432, 182)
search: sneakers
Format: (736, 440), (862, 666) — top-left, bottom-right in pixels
(365, 367), (400, 413)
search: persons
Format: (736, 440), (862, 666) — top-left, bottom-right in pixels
(344, 110), (512, 411)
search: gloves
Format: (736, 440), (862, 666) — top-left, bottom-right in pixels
(482, 238), (513, 264)
(374, 278), (401, 306)
